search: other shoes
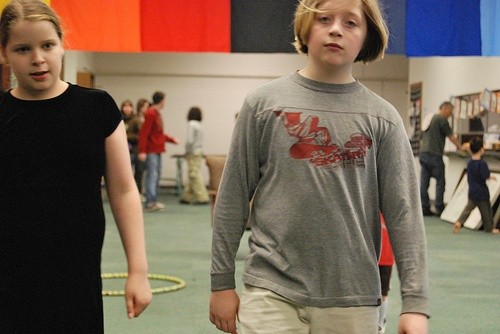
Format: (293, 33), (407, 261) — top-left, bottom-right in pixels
(143, 201), (165, 212)
(422, 206), (435, 216)
(192, 201), (209, 205)
(179, 198), (190, 204)
(377, 315), (387, 334)
(436, 204), (446, 214)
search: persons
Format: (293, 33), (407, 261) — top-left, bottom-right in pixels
(420, 101), (469, 217)
(466, 109), (488, 143)
(376, 210), (395, 334)
(121, 91), (178, 211)
(208, 0), (431, 334)
(0, 0), (152, 334)
(450, 136), (500, 233)
(179, 106), (210, 205)
